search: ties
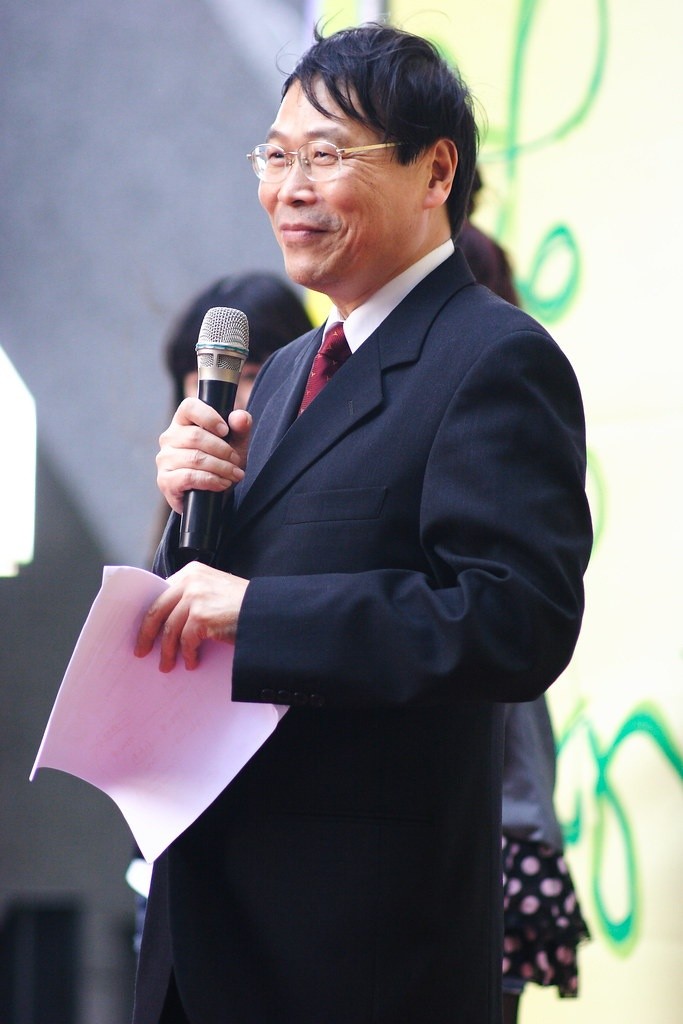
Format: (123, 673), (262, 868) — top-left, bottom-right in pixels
(297, 323), (352, 437)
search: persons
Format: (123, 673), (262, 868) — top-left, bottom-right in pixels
(456, 225), (594, 1022)
(163, 269), (314, 419)
(133, 21), (593, 1024)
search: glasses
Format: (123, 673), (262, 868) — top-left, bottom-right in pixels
(246, 141), (404, 182)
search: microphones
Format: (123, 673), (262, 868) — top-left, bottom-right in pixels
(177, 307), (251, 558)
